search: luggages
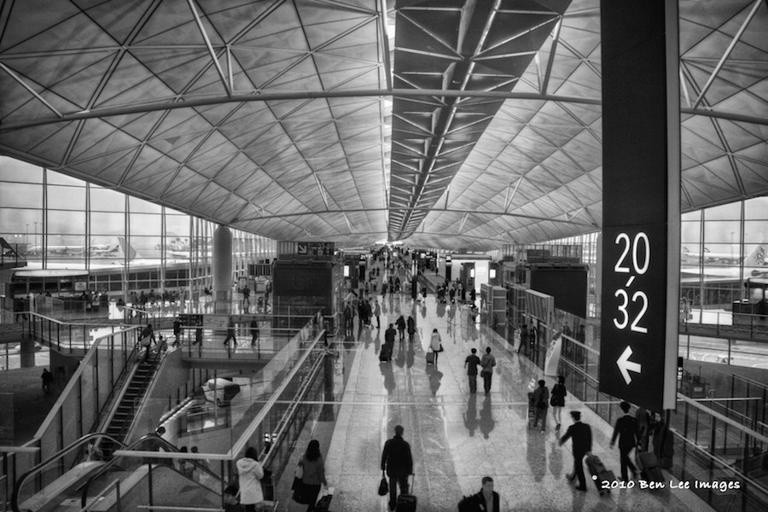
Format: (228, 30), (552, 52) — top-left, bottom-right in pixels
(427, 346), (434, 365)
(640, 467), (664, 485)
(397, 473), (417, 512)
(596, 470), (616, 495)
(380, 350), (391, 361)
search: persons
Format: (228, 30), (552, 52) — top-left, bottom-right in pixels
(10, 244), (654, 512)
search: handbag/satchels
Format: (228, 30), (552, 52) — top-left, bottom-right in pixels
(440, 344), (443, 352)
(637, 450), (659, 470)
(290, 462), (303, 490)
(140, 335), (151, 346)
(378, 478), (389, 496)
(480, 371), (484, 377)
(315, 495), (332, 511)
(382, 345), (391, 350)
(586, 455), (606, 476)
